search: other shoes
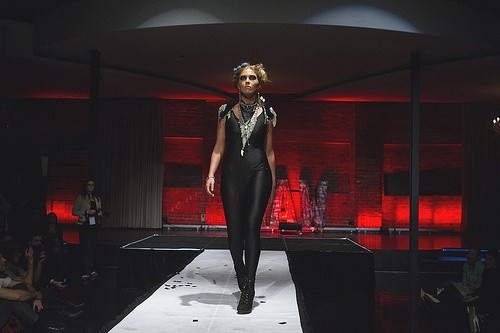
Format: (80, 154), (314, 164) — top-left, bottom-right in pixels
(81, 274), (89, 281)
(90, 271), (98, 279)
(68, 310), (82, 322)
(45, 326), (67, 333)
(70, 303), (85, 310)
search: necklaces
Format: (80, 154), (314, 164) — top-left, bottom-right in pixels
(238, 99), (260, 146)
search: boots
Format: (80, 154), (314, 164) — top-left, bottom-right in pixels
(235, 267), (246, 291)
(238, 271), (255, 314)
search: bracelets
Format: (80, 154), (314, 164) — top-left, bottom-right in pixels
(206, 175), (215, 181)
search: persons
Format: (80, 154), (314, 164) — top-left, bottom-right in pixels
(71, 178), (105, 282)
(459, 242), (486, 308)
(0, 210), (99, 332)
(205, 61), (278, 316)
(471, 247), (500, 333)
